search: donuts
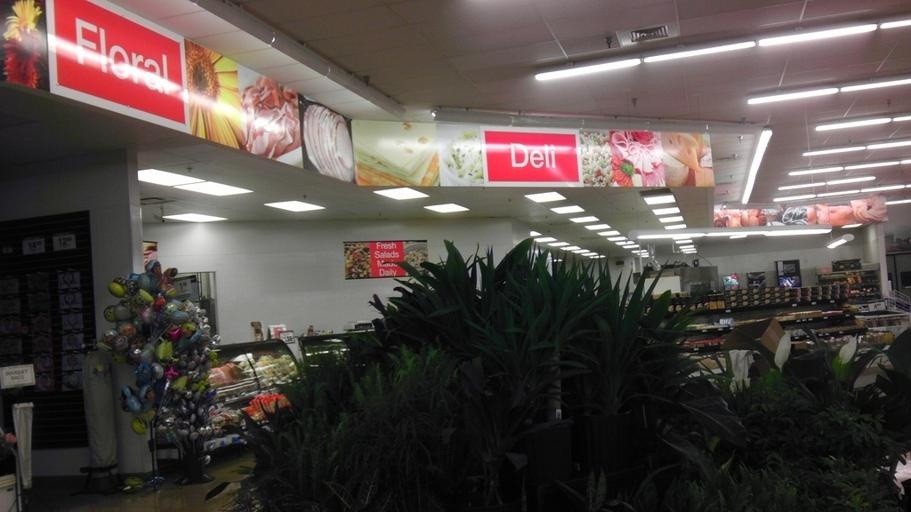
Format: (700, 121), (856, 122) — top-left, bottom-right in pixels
(818, 204), (853, 227)
(853, 196), (886, 224)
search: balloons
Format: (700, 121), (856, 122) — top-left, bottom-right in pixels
(96, 260), (221, 464)
